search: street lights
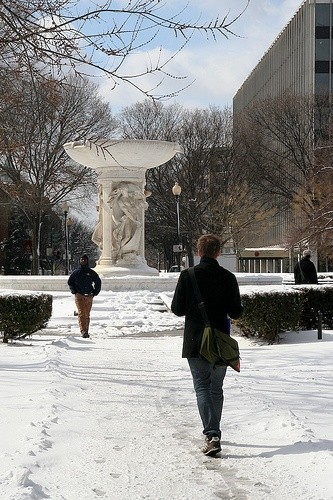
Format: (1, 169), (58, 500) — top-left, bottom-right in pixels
(172, 181), (181, 272)
(62, 202), (69, 275)
(67, 218), (72, 272)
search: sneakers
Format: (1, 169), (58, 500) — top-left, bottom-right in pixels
(203, 437), (221, 455)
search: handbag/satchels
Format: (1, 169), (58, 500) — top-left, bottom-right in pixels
(200, 325), (240, 372)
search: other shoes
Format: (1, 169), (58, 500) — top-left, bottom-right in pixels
(82, 333), (89, 337)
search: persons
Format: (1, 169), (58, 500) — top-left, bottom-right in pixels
(92, 206), (102, 246)
(171, 234), (244, 453)
(294, 251), (318, 284)
(67, 254), (101, 338)
(118, 184), (149, 247)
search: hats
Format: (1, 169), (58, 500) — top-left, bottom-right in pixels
(303, 250), (311, 257)
